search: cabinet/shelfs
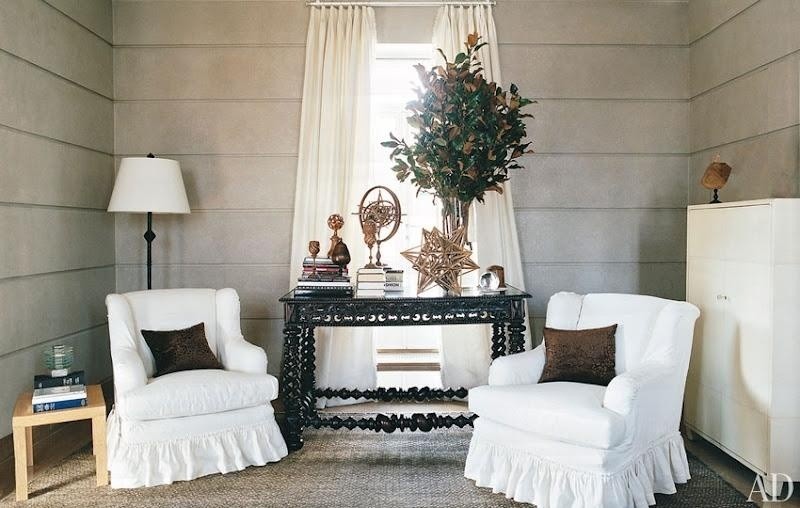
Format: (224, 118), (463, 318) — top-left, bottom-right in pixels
(681, 197), (800, 497)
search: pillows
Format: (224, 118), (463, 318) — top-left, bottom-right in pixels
(536, 324), (620, 386)
(139, 322), (225, 378)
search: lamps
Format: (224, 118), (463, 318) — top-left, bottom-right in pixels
(106, 152), (191, 289)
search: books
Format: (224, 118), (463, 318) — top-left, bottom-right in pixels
(357, 266), (404, 298)
(31, 373), (89, 413)
(300, 257), (352, 281)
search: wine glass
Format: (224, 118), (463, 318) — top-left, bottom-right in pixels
(308, 241), (321, 280)
(333, 241), (350, 282)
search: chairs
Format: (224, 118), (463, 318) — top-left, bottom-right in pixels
(461, 290), (704, 508)
(104, 287), (289, 493)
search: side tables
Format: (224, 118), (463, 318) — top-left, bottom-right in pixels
(11, 384), (108, 503)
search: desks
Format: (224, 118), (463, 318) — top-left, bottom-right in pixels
(278, 280), (534, 451)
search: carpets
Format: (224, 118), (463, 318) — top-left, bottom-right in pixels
(0, 410), (765, 508)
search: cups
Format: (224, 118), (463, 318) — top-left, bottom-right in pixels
(386, 269), (404, 291)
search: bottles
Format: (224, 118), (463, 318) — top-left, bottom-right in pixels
(487, 265), (507, 289)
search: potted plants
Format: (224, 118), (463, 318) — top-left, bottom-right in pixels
(379, 31), (541, 258)
